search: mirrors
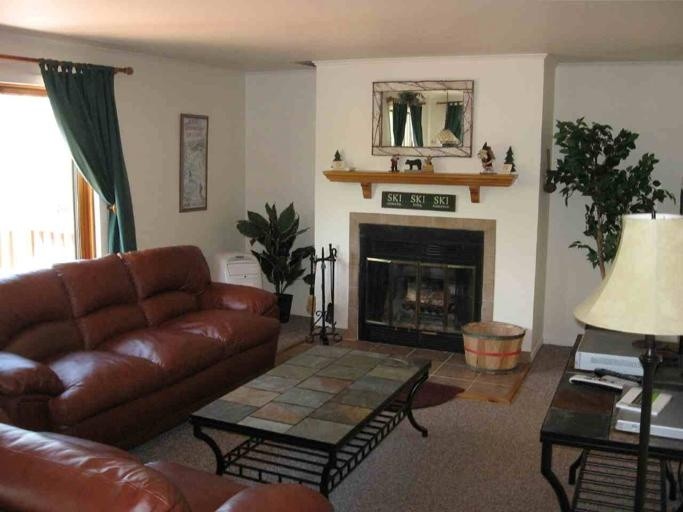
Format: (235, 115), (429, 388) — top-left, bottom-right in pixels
(372, 79), (473, 158)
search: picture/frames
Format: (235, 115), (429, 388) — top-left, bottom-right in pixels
(178, 111), (210, 212)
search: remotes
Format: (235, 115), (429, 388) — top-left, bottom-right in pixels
(569, 368), (641, 393)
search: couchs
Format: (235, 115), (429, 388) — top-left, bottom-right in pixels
(0, 244), (280, 449)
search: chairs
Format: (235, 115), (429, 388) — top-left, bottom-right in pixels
(0, 424), (335, 512)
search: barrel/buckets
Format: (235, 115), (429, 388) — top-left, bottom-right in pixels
(460, 322), (527, 374)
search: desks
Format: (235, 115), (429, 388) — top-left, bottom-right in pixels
(540, 330), (683, 510)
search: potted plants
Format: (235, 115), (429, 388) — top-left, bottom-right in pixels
(234, 200), (315, 324)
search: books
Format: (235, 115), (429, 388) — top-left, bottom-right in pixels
(616, 385), (674, 417)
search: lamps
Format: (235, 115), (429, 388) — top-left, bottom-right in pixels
(571, 211), (683, 512)
